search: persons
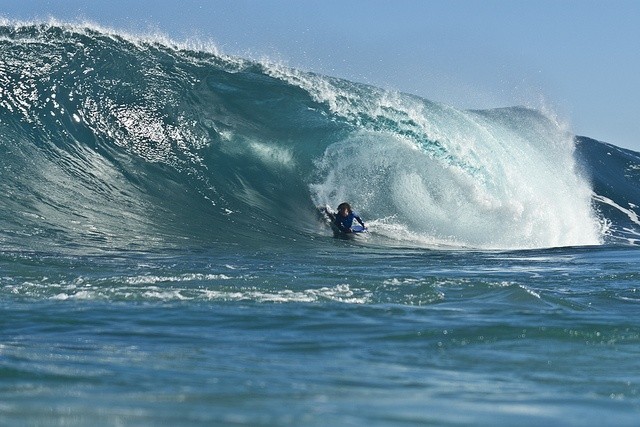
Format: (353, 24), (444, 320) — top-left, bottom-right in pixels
(334, 203), (365, 232)
